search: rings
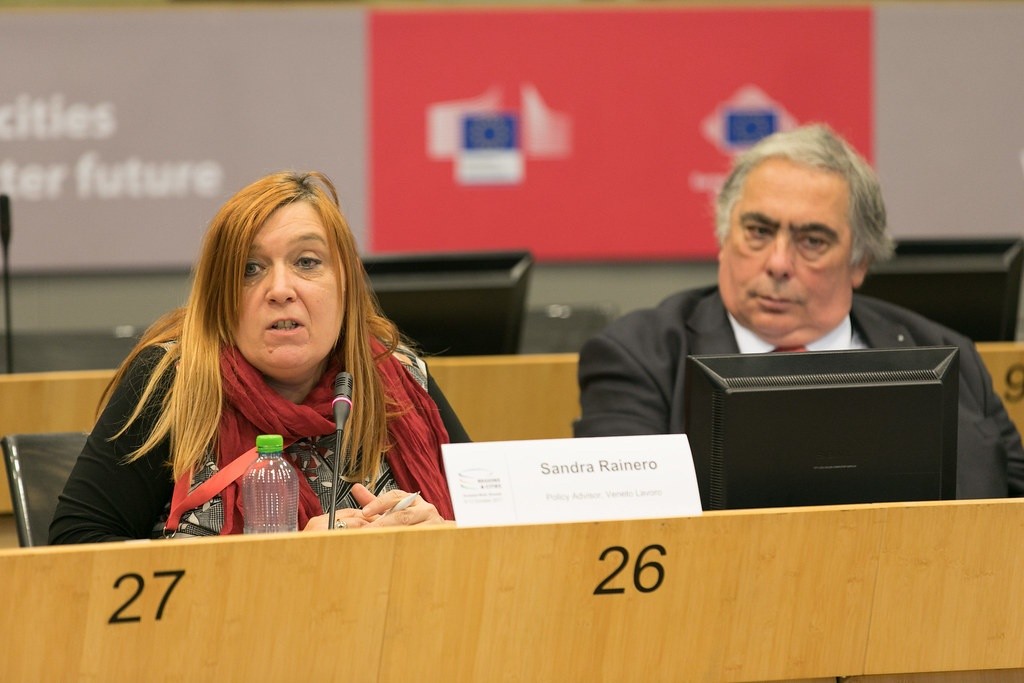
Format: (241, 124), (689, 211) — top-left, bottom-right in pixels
(336, 518), (347, 529)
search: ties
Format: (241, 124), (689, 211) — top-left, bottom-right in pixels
(775, 347), (807, 352)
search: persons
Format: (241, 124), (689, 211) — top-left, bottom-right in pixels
(49, 169), (473, 547)
(572, 123), (1024, 511)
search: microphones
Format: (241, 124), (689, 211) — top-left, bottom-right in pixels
(328, 372), (353, 531)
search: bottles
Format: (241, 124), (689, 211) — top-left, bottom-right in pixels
(242, 433), (300, 532)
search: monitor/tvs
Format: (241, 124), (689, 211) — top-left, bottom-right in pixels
(683, 344), (960, 511)
(355, 249), (533, 358)
